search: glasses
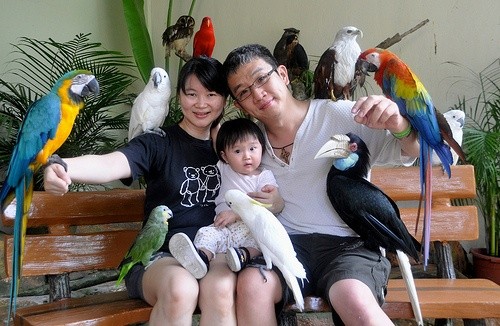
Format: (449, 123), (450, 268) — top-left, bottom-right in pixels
(234, 67), (276, 102)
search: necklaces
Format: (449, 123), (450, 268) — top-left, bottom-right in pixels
(272, 143), (293, 165)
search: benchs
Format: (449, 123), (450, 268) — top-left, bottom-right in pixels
(0, 164), (500, 326)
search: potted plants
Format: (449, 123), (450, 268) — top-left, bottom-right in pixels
(440, 57), (500, 285)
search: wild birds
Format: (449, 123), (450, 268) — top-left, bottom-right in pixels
(272, 27), (309, 86)
(314, 132), (427, 264)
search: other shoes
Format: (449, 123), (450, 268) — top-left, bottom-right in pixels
(226, 246), (246, 272)
(168, 233), (210, 279)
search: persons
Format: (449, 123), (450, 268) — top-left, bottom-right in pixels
(168, 118), (279, 280)
(222, 43), (420, 326)
(44, 56), (285, 326)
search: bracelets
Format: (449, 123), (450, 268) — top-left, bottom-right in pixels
(392, 123), (413, 141)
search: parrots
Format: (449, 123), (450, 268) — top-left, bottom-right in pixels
(224, 189), (309, 312)
(0, 69), (100, 326)
(128, 67), (171, 142)
(355, 48), (470, 272)
(113, 205), (173, 288)
